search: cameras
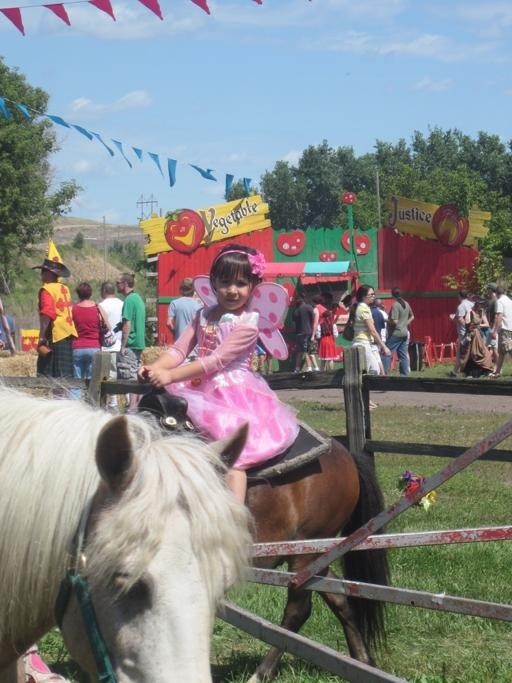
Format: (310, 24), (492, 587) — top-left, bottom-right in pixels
(113, 321), (124, 333)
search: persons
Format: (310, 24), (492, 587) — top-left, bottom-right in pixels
(69, 281), (102, 379)
(291, 287), (389, 376)
(135, 239), (302, 512)
(96, 282), (125, 409)
(254, 338), (275, 376)
(445, 279), (512, 380)
(379, 287), (416, 376)
(162, 276), (205, 362)
(33, 262), (74, 377)
(344, 285), (391, 412)
(115, 272), (147, 411)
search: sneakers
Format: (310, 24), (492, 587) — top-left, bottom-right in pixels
(445, 372), (456, 378)
(254, 367), (319, 377)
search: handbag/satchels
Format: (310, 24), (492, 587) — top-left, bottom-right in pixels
(96, 303), (117, 346)
(344, 317), (354, 340)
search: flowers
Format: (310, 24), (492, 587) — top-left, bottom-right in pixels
(398, 467), (439, 510)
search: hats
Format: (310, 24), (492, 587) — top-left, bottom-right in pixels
(487, 282), (497, 289)
(474, 300), (487, 305)
(179, 276), (194, 294)
(32, 259), (71, 277)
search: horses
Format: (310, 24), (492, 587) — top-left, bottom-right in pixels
(131, 366), (394, 659)
(3, 372), (259, 658)
